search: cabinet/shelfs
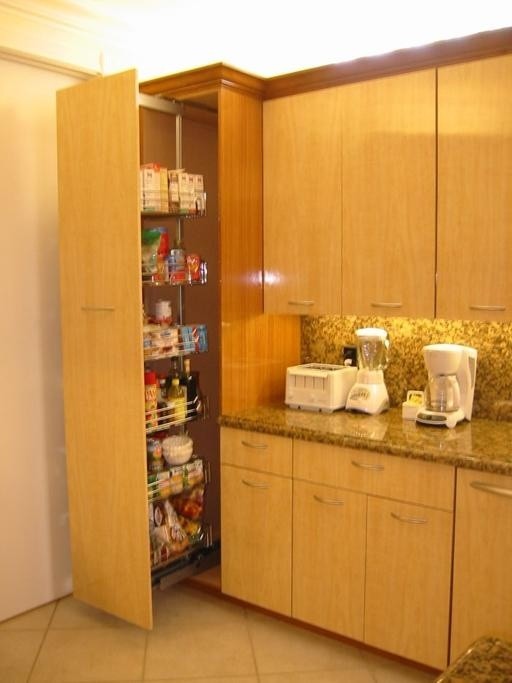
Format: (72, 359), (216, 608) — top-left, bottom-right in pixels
(55, 63), (302, 635)
(449, 466), (512, 669)
(219, 425), (292, 624)
(293, 438), (458, 674)
(438, 51), (512, 324)
(340, 66), (437, 320)
(262, 66), (339, 316)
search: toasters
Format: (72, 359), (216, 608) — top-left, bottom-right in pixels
(283, 360), (357, 413)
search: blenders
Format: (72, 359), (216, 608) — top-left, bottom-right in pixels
(346, 326), (392, 415)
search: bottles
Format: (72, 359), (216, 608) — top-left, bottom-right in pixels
(145, 356), (200, 427)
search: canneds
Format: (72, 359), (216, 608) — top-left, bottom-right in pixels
(147, 438), (164, 472)
(169, 248), (186, 282)
(186, 254), (202, 282)
(150, 226), (169, 261)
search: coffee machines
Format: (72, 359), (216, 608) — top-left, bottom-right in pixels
(416, 342), (479, 428)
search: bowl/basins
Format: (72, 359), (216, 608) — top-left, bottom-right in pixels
(161, 434), (195, 464)
